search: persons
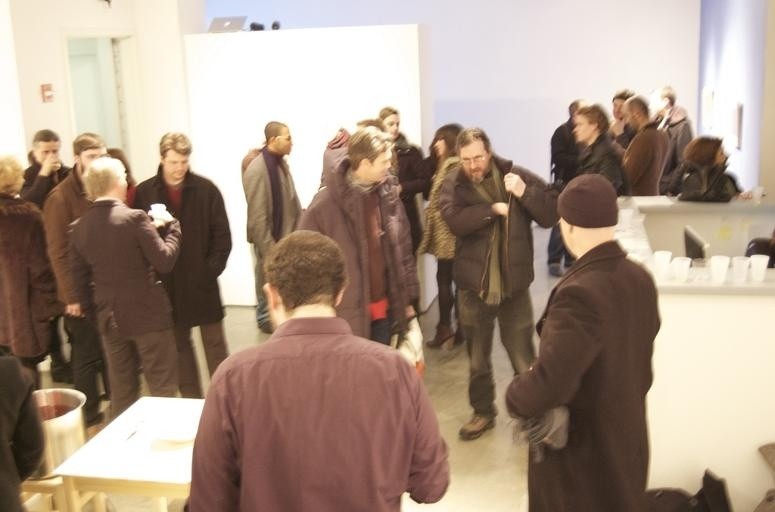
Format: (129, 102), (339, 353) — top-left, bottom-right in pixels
(0, 129), (231, 512)
(299, 106), (562, 441)
(494, 172), (661, 512)
(548, 86), (741, 277)
(242, 122), (303, 337)
(181, 230), (451, 511)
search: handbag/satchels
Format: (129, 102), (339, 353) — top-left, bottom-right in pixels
(398, 316), (427, 381)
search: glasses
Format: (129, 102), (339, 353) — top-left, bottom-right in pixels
(272, 134), (292, 143)
(461, 154), (489, 165)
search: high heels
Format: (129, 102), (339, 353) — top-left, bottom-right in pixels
(452, 322), (466, 347)
(427, 321), (456, 351)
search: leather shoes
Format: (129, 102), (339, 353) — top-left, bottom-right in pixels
(51, 364), (76, 384)
(85, 411), (105, 427)
(549, 266), (565, 278)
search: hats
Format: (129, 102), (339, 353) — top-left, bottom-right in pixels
(556, 172), (620, 228)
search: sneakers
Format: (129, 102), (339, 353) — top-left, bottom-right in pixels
(458, 414), (497, 441)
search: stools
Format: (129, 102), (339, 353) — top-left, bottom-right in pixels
(20, 475), (66, 512)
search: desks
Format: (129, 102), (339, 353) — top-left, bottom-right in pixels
(51, 396), (205, 512)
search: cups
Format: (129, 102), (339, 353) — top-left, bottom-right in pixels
(710, 255), (732, 284)
(615, 206), (651, 253)
(751, 252), (769, 280)
(670, 258), (687, 285)
(733, 255), (750, 281)
(629, 246), (671, 286)
(691, 256), (706, 282)
